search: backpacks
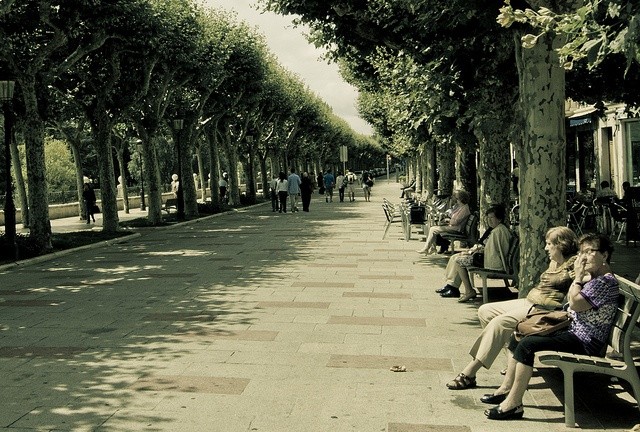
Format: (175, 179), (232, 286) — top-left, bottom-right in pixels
(347, 174), (354, 184)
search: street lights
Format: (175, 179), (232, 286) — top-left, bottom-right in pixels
(0, 78), (20, 262)
(173, 116), (184, 219)
(137, 139), (146, 210)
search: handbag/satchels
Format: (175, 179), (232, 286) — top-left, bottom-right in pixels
(410, 206), (425, 225)
(516, 304), (573, 341)
(331, 182), (336, 188)
(342, 180), (348, 187)
(473, 253), (484, 267)
(366, 180), (374, 188)
(86, 205), (101, 215)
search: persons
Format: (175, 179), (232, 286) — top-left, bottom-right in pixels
(323, 170), (334, 203)
(454, 207), (512, 303)
(596, 181), (615, 197)
(446, 226), (581, 390)
(359, 168), (372, 202)
(435, 204), (507, 297)
(621, 182), (630, 198)
(82, 183), (96, 224)
(270, 174), (279, 212)
(435, 190), (464, 253)
(479, 232), (620, 420)
(336, 172), (344, 202)
(416, 191), (471, 256)
(288, 168), (302, 211)
(399, 177), (416, 197)
(302, 172), (312, 212)
(344, 168), (357, 202)
(278, 172), (288, 213)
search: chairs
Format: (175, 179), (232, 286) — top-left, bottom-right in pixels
(609, 202), (627, 241)
(401, 198), (429, 242)
(425, 192), (451, 226)
(597, 195), (616, 233)
(380, 197), (404, 240)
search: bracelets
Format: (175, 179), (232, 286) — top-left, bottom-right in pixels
(575, 282), (583, 287)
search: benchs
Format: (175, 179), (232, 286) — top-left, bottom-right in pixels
(440, 215), (476, 255)
(162, 198), (177, 213)
(468, 230), (520, 304)
(534, 273), (640, 427)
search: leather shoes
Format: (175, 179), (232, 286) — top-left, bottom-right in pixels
(440, 290), (460, 297)
(435, 284), (453, 293)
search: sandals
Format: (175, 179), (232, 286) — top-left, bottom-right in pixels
(480, 391), (509, 404)
(446, 373), (476, 389)
(484, 404), (523, 420)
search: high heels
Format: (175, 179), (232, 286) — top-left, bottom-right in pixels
(458, 290), (476, 302)
(437, 246), (448, 253)
(417, 250), (427, 256)
(427, 249), (437, 255)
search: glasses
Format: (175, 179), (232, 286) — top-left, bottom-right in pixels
(577, 248), (598, 256)
